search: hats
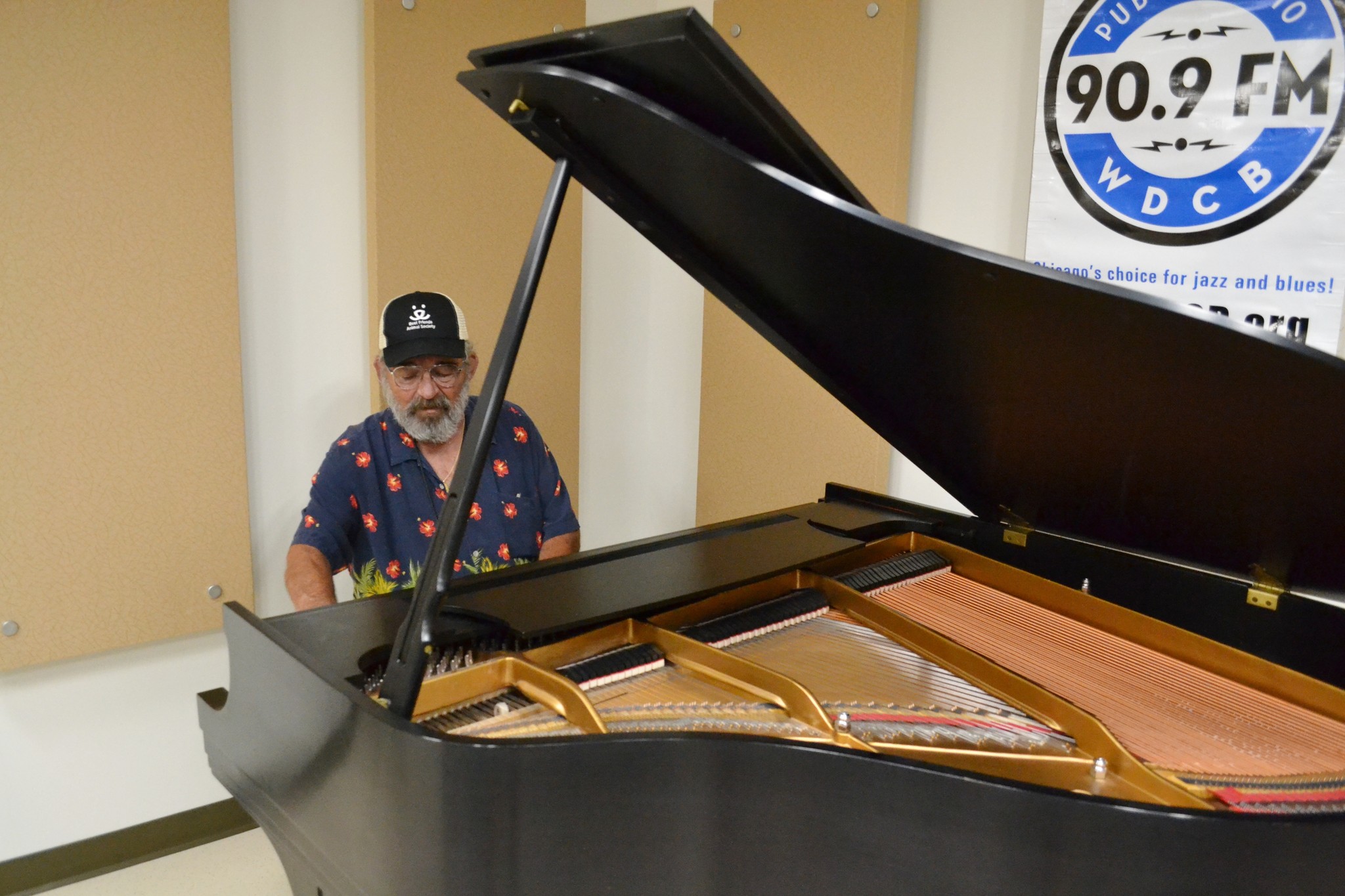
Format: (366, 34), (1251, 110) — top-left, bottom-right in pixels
(377, 291), (468, 367)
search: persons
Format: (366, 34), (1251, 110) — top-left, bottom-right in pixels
(285, 292), (580, 612)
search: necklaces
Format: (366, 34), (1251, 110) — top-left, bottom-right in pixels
(443, 457), (457, 493)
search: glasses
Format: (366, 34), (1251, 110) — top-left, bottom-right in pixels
(386, 351), (468, 390)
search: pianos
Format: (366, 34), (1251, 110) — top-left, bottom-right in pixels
(196, 9), (1345, 895)
